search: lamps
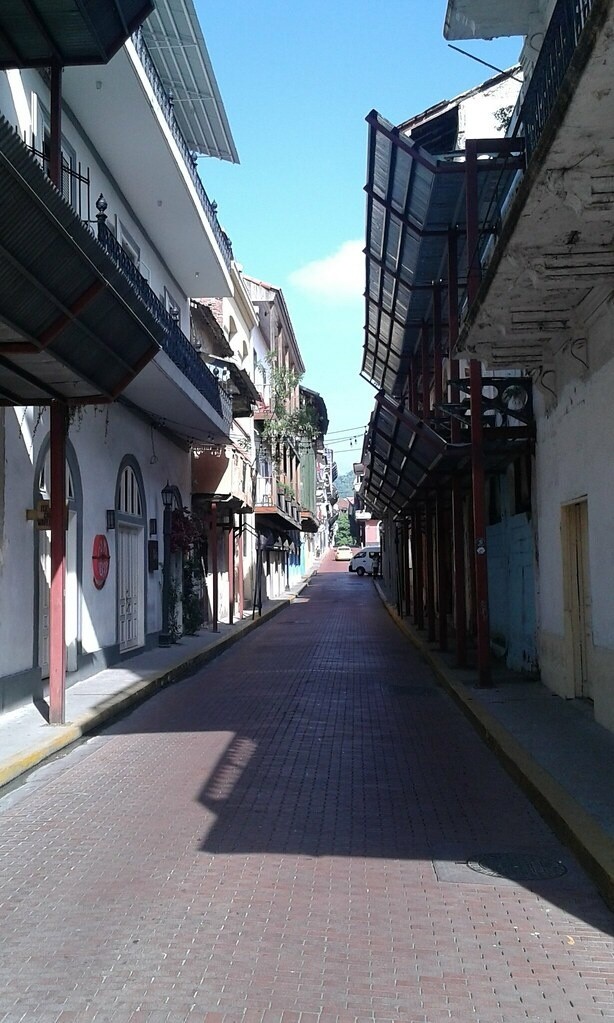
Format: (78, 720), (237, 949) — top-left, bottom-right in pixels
(150, 518), (157, 535)
(195, 341), (201, 349)
(106, 509), (115, 530)
(170, 305), (179, 316)
(161, 479), (174, 512)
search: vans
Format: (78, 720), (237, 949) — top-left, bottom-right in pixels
(348, 546), (381, 576)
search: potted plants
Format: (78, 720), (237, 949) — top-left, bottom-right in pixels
(276, 481), (295, 502)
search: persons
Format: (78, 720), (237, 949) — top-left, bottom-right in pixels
(370, 556), (379, 580)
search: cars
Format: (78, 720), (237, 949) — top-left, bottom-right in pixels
(335, 546), (354, 561)
